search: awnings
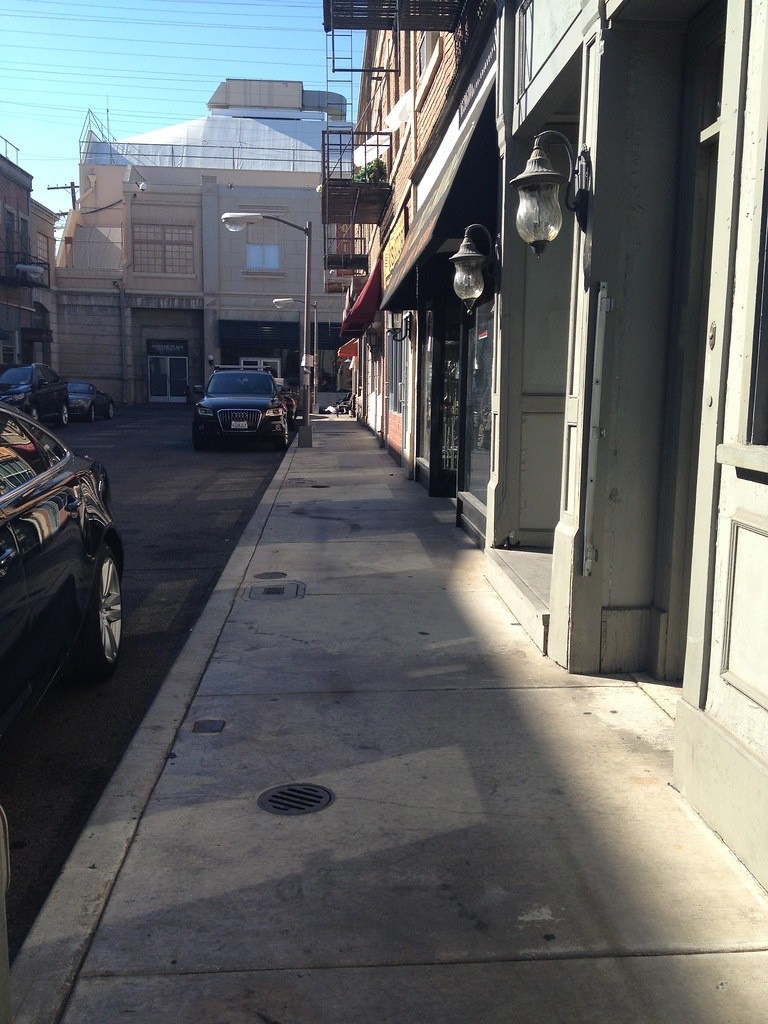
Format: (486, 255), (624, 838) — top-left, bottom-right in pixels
(338, 338), (357, 357)
(338, 261), (381, 338)
(379, 74), (500, 313)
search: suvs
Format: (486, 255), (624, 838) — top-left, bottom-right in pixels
(0, 362), (71, 426)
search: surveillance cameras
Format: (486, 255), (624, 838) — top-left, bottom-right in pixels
(139, 183), (147, 192)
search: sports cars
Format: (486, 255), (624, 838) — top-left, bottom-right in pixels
(64, 381), (114, 422)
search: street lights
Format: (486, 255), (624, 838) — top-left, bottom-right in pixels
(221, 212), (314, 449)
(272, 298), (319, 415)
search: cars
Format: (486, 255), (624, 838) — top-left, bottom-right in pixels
(0, 402), (125, 773)
(269, 369), (296, 425)
(443, 382), (492, 448)
(192, 365), (291, 450)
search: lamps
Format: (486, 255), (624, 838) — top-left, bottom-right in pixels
(449, 222), (503, 316)
(386, 312), (412, 344)
(509, 128), (589, 258)
